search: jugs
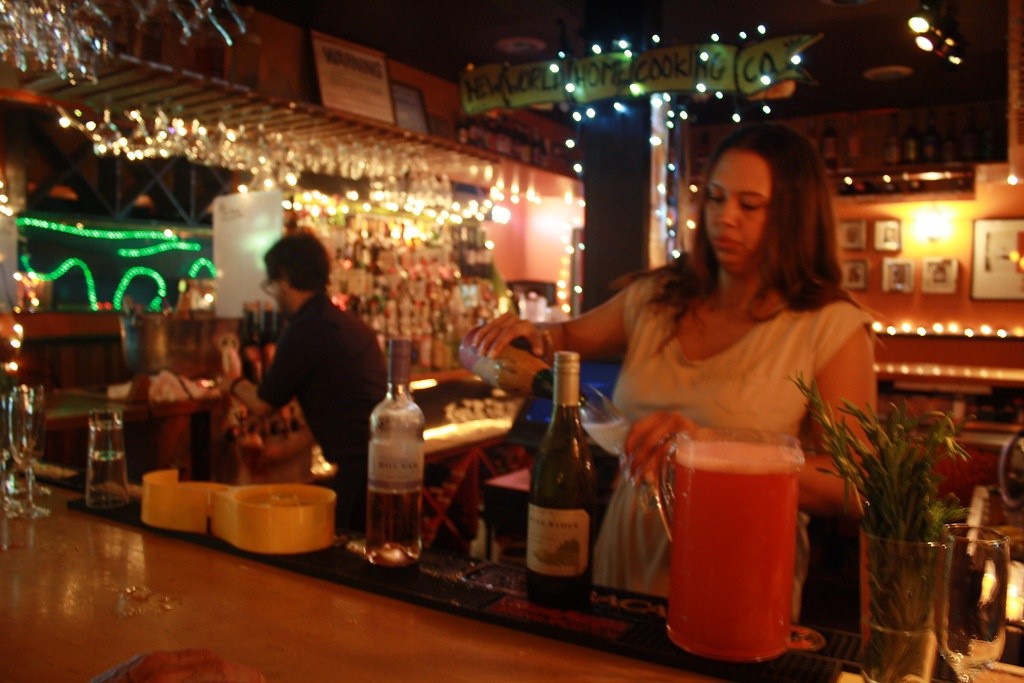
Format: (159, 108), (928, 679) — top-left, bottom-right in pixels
(654, 426), (806, 664)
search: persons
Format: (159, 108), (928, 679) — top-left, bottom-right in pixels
(469, 127), (878, 621)
(247, 231), (390, 537)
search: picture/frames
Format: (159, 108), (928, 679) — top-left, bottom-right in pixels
(835, 217), (960, 294)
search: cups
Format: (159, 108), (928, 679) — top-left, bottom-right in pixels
(14, 273), (54, 313)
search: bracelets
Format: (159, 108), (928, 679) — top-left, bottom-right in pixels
(537, 324), (554, 350)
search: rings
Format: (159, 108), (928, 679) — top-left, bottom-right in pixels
(663, 432), (679, 455)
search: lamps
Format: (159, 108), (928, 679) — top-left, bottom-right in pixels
(908, 0), (967, 65)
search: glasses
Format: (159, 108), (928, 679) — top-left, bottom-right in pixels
(260, 278), (280, 297)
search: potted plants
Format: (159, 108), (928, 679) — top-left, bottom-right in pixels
(786, 370), (973, 683)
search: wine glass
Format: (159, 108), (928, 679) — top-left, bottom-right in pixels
(0, 383), (50, 549)
(576, 381), (671, 507)
(94, 97), (537, 219)
(932, 524), (1011, 683)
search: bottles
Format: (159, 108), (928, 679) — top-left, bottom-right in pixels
(843, 171), (973, 195)
(696, 129), (713, 176)
(244, 192), (555, 404)
(882, 103), (1007, 167)
(173, 278), (218, 319)
(364, 338), (426, 568)
(83, 407), (131, 512)
(455, 108), (562, 170)
(107, 0), (232, 81)
(804, 112), (865, 171)
(524, 350), (594, 604)
